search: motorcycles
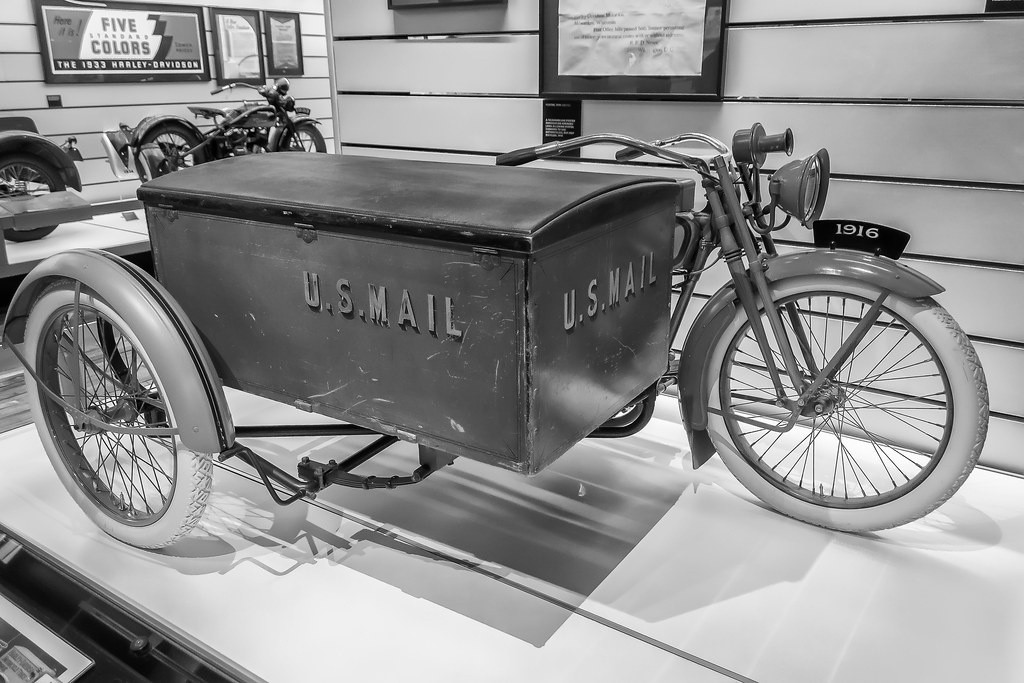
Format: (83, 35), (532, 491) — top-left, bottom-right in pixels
(1, 125), (984, 558)
(0, 130), (84, 242)
(102, 78), (327, 186)
